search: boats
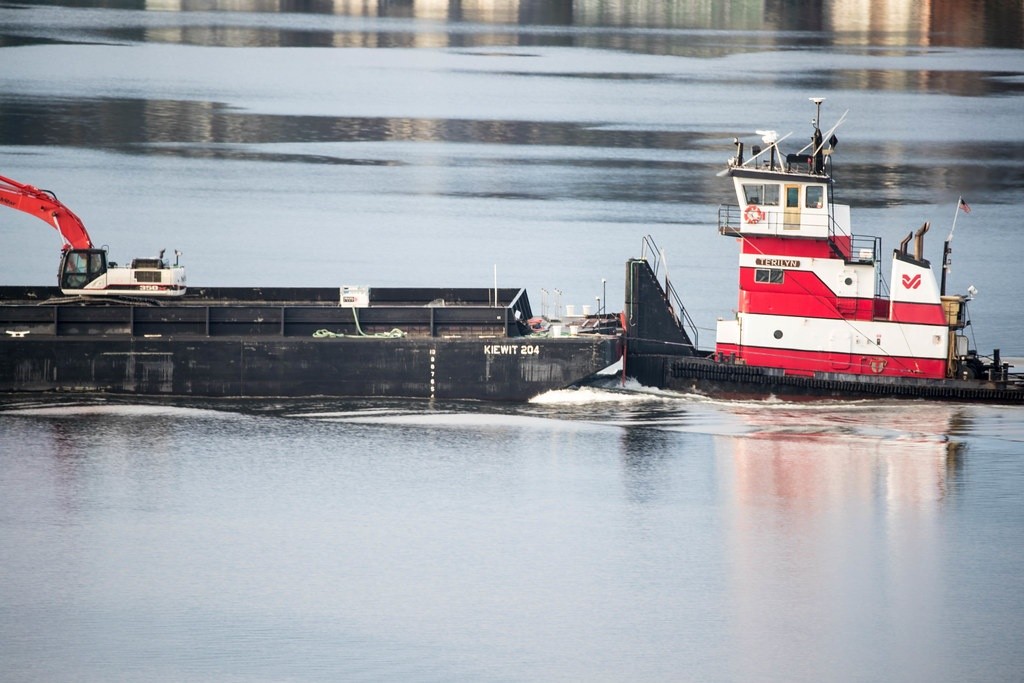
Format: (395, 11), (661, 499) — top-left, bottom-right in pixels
(0, 283), (624, 412)
(624, 97), (1024, 407)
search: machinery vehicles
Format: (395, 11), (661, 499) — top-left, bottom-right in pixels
(0, 173), (189, 298)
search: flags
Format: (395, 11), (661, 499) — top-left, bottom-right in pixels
(960, 198), (971, 213)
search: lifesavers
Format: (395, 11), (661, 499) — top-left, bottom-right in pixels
(744, 205), (761, 224)
